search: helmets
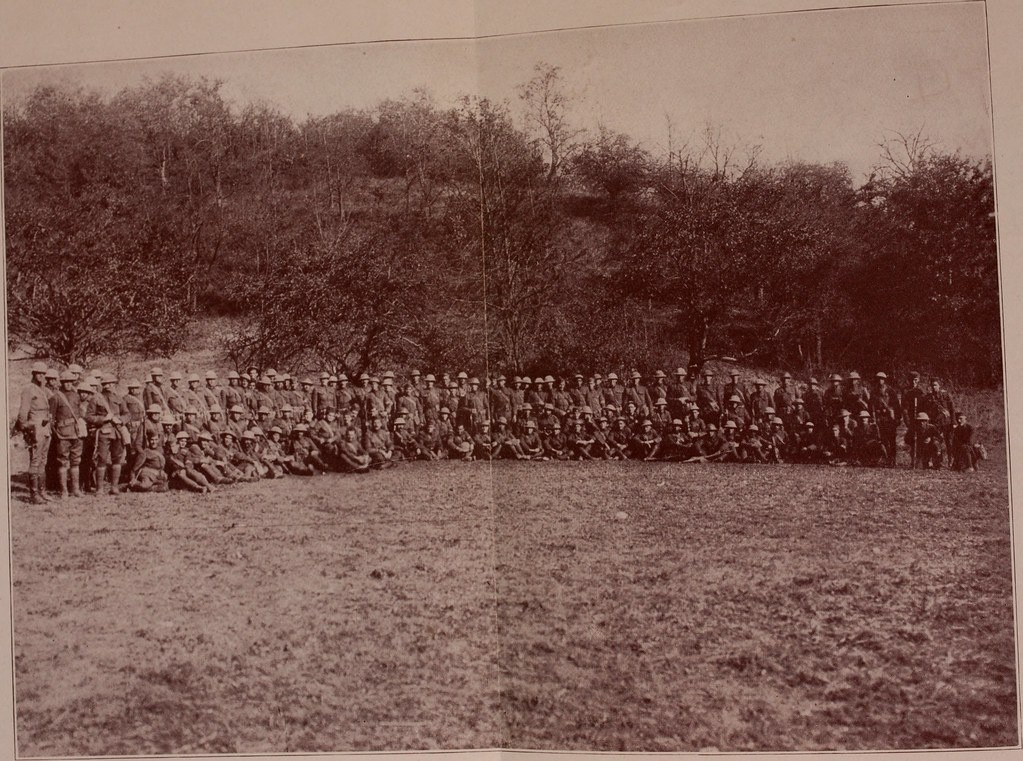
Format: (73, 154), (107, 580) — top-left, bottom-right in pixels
(22, 362), (987, 434)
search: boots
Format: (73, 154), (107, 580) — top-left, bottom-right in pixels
(28, 472), (46, 504)
(58, 467), (69, 500)
(111, 464), (121, 494)
(71, 466), (86, 497)
(38, 473), (52, 500)
(94, 467), (106, 494)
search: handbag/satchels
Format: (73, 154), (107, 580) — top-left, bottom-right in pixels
(973, 443), (987, 459)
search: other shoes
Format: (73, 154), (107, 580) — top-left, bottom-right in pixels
(194, 469), (284, 494)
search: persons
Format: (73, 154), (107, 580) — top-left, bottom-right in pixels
(14, 362), (54, 504)
(774, 371), (900, 468)
(925, 378), (956, 467)
(950, 413), (982, 473)
(45, 363), (788, 497)
(905, 411), (947, 469)
(901, 371), (926, 427)
